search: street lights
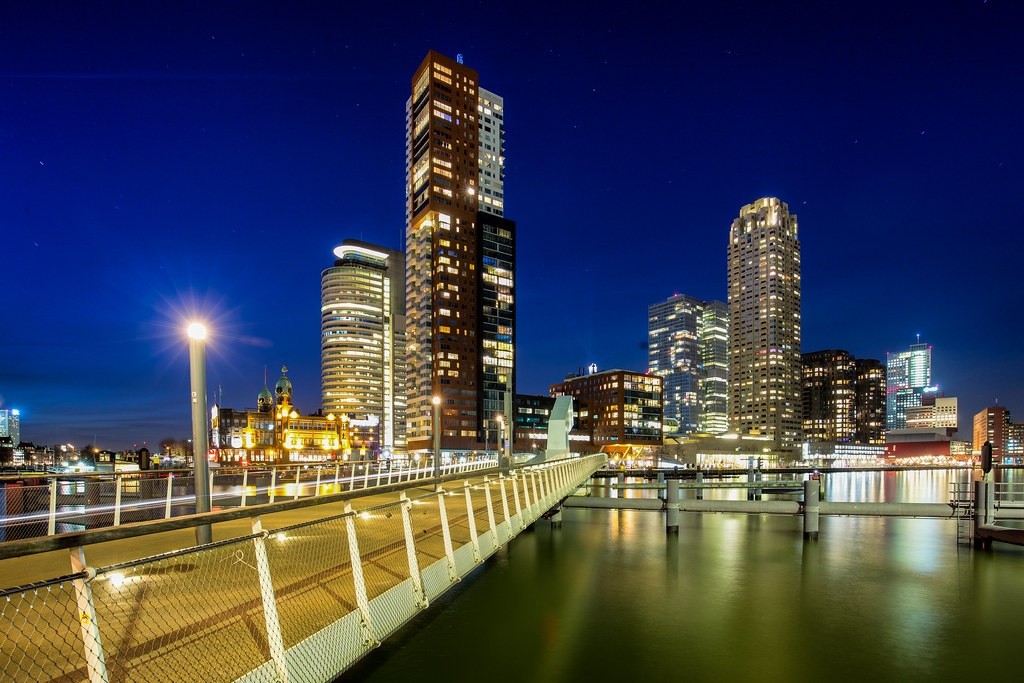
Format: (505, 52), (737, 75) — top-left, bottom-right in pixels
(432, 396), (442, 493)
(188, 321), (216, 546)
(497, 415), (503, 476)
